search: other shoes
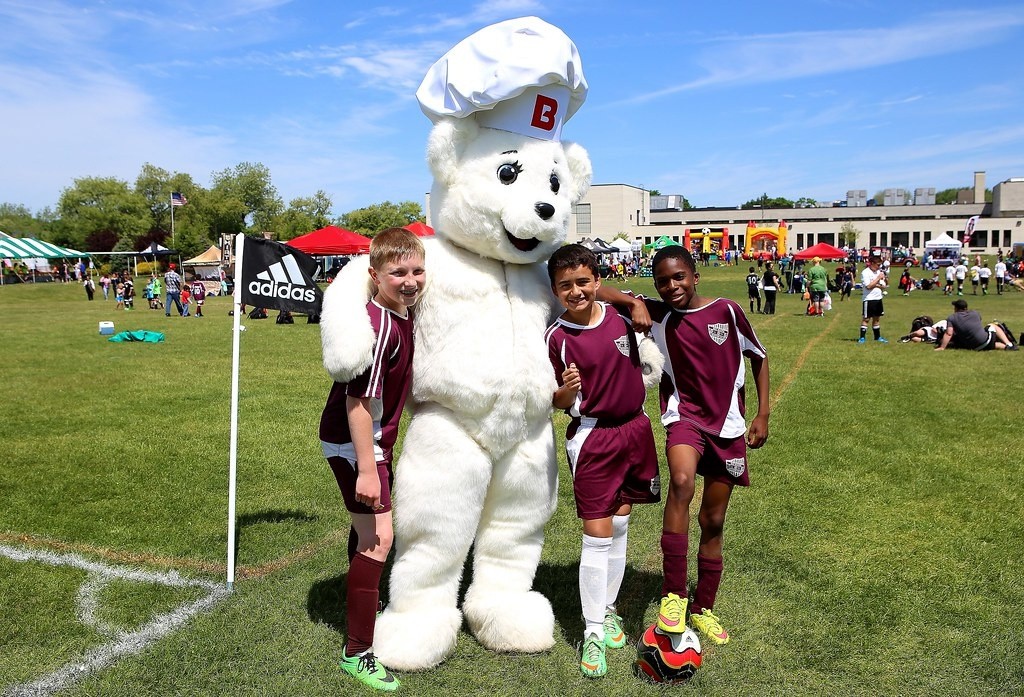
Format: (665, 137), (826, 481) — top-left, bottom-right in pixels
(857, 337), (865, 343)
(1005, 343), (1019, 350)
(166, 313), (170, 316)
(944, 286), (1004, 295)
(877, 337), (888, 343)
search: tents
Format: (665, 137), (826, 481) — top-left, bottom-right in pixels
(182, 245), (235, 285)
(284, 222), (434, 255)
(645, 236), (683, 251)
(0, 237), (92, 263)
(925, 233), (963, 267)
(789, 242), (848, 294)
(579, 237), (634, 254)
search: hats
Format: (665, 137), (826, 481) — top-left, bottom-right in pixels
(812, 256), (822, 263)
(169, 263), (177, 269)
(952, 300), (967, 310)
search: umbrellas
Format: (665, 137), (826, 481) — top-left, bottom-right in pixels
(140, 242), (178, 279)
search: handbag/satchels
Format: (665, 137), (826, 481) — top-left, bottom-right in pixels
(823, 293), (831, 310)
(98, 281), (105, 287)
(128, 289), (136, 296)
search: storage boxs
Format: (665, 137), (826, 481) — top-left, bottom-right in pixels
(99, 321), (114, 334)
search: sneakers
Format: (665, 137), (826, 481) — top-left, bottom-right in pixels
(338, 645), (401, 691)
(604, 610), (626, 648)
(689, 607), (729, 645)
(657, 593), (688, 633)
(581, 639), (607, 677)
(375, 610), (382, 618)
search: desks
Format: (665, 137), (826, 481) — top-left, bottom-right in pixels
(202, 281), (232, 296)
(934, 259), (953, 265)
(35, 272), (55, 281)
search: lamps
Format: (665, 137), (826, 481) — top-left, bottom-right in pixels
(789, 225), (792, 228)
(1017, 221), (1021, 224)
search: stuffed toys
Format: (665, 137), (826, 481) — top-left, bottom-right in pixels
(319, 16), (664, 671)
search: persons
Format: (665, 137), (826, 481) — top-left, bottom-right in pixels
(595, 246), (769, 645)
(857, 256), (888, 343)
(901, 300), (1019, 351)
(320, 228), (426, 690)
(692, 245), (745, 267)
(897, 243), (992, 296)
(746, 245), (891, 316)
(995, 248), (1024, 295)
(545, 244), (661, 678)
(51, 261), (85, 283)
(596, 254), (653, 283)
(83, 263), (206, 317)
(1, 261), (30, 283)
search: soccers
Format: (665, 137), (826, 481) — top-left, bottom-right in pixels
(635, 624), (702, 684)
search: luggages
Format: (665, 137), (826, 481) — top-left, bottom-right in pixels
(900, 334), (912, 342)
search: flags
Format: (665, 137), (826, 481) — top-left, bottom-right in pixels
(232, 234), (324, 317)
(172, 192), (187, 206)
